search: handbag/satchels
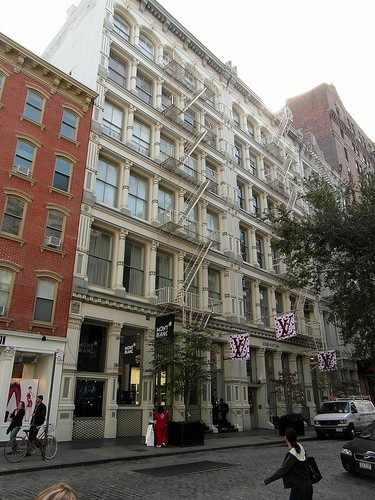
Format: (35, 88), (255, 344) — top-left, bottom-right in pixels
(307, 457), (322, 483)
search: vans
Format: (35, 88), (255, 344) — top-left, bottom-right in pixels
(312, 395), (375, 440)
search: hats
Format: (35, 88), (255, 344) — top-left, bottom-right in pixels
(37, 395), (43, 400)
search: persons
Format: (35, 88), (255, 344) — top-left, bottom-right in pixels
(153, 405), (170, 447)
(4, 378), (21, 422)
(25, 394), (46, 456)
(213, 398), (229, 430)
(34, 482), (78, 500)
(262, 428), (313, 500)
(25, 386), (35, 422)
(7, 401), (25, 455)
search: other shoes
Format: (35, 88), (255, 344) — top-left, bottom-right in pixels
(215, 425), (219, 427)
(15, 449), (19, 452)
(6, 451), (12, 455)
(22, 453), (31, 456)
(162, 443), (166, 448)
(156, 445), (161, 447)
(222, 427), (228, 429)
(41, 445), (45, 456)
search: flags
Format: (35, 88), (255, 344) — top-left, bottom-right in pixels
(230, 333), (251, 360)
(318, 350), (338, 372)
(274, 313), (298, 340)
(155, 312), (175, 355)
(123, 335), (136, 364)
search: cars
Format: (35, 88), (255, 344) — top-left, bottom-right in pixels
(339, 419), (375, 480)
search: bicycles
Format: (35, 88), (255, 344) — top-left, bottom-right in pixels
(4, 423), (58, 463)
